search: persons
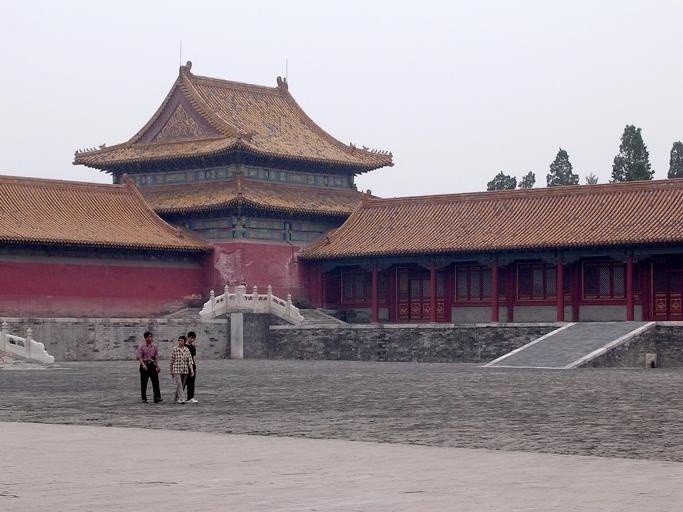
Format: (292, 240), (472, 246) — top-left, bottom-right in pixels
(134, 331), (162, 403)
(174, 331), (198, 403)
(169, 336), (194, 404)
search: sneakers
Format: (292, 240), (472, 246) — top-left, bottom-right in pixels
(177, 400), (185, 404)
(186, 399), (198, 404)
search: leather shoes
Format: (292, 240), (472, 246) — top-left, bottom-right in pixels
(154, 398), (163, 403)
(142, 400), (149, 403)
